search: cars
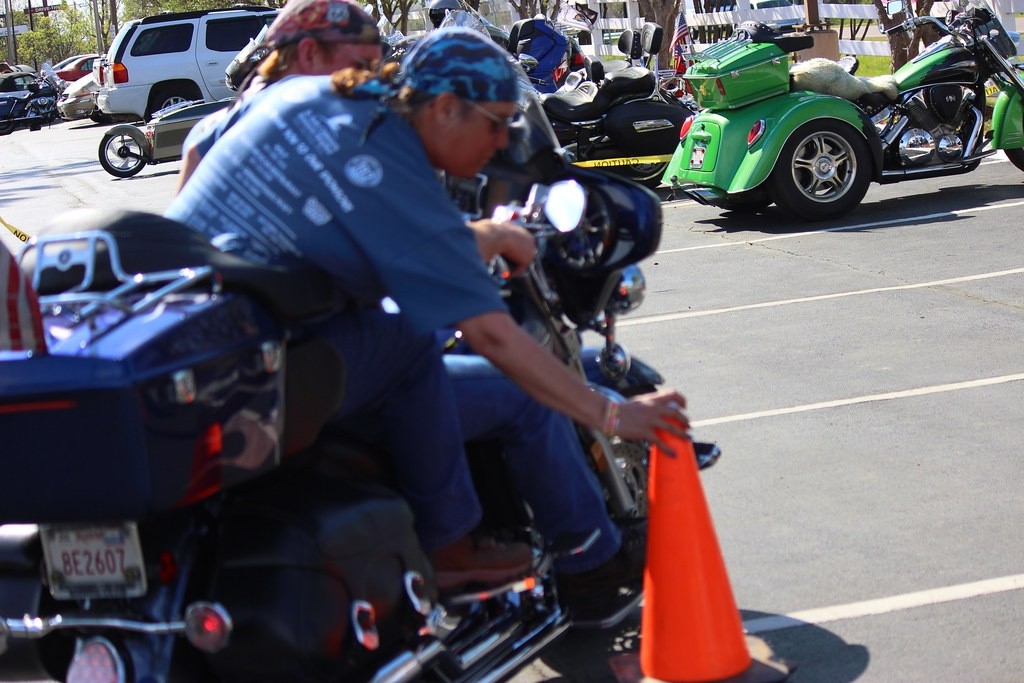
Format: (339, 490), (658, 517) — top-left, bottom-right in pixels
(0, 54), (97, 135)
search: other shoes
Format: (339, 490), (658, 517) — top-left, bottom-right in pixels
(557, 541), (630, 608)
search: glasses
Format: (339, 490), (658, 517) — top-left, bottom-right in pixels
(463, 101), (512, 134)
(329, 48), (370, 71)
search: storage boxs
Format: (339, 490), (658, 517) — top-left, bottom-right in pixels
(682, 40), (790, 111)
(0, 290), (263, 530)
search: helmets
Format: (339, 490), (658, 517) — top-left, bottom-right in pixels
(428, 0), (463, 29)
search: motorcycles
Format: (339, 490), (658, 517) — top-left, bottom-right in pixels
(656, 0), (1024, 227)
(99, 1), (705, 195)
(1, 25), (725, 683)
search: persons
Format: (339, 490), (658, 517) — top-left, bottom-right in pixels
(161, 0), (693, 605)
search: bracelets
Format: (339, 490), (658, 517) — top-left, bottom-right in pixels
(602, 395), (622, 437)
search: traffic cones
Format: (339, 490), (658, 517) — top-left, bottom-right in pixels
(608, 402), (799, 683)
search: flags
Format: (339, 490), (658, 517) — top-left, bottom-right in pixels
(669, 12), (690, 54)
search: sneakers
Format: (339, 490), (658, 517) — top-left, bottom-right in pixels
(431, 530), (533, 588)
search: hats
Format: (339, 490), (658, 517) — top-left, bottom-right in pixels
(396, 26), (522, 101)
(265, 0), (390, 52)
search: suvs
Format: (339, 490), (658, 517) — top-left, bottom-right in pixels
(86, 4), (284, 125)
